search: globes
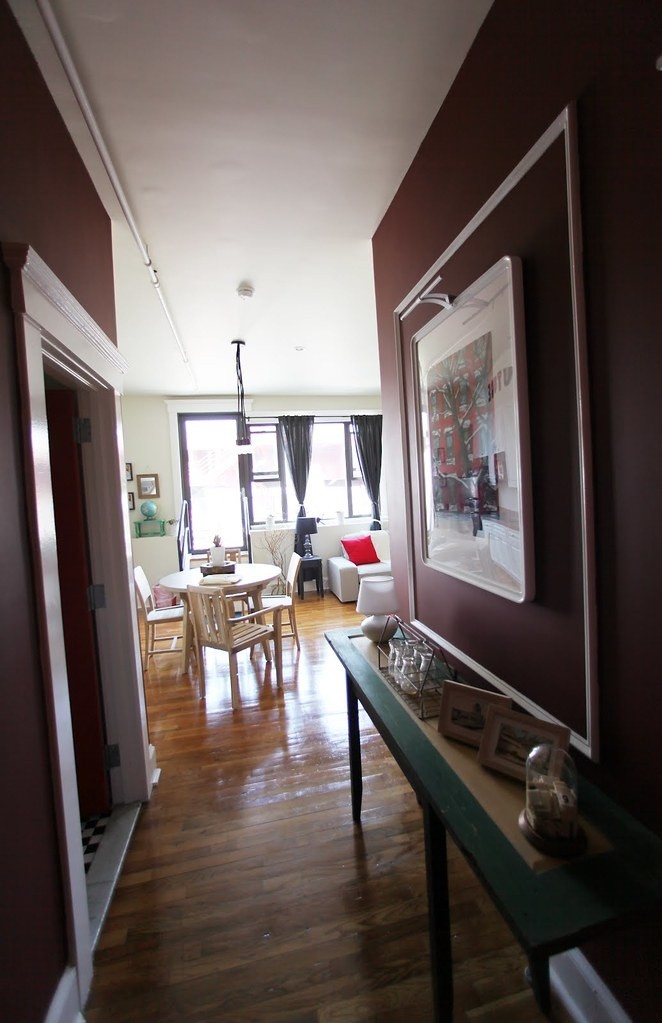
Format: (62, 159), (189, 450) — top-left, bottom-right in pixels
(141, 500), (158, 521)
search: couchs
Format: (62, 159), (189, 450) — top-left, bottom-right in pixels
(326, 530), (391, 603)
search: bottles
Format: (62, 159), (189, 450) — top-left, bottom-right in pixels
(387, 638), (437, 695)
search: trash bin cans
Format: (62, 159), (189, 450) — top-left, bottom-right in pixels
(153, 584), (177, 610)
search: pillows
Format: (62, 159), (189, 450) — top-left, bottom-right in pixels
(340, 535), (381, 566)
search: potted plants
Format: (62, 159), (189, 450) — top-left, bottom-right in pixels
(210, 535), (225, 566)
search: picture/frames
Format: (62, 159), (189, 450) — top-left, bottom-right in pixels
(128, 492), (135, 510)
(477, 703), (570, 787)
(409, 254), (535, 605)
(436, 679), (511, 748)
(136, 473), (160, 499)
(126, 462), (133, 481)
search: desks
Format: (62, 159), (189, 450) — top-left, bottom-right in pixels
(159, 563), (282, 672)
(132, 519), (166, 538)
(323, 626), (662, 1023)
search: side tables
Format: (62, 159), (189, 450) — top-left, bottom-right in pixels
(297, 555), (324, 599)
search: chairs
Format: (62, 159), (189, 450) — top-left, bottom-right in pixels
(134, 566), (186, 672)
(207, 549), (251, 631)
(247, 551), (302, 661)
(186, 585), (283, 711)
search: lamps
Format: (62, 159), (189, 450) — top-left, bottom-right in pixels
(356, 575), (400, 643)
(295, 517), (318, 559)
(232, 339), (255, 456)
